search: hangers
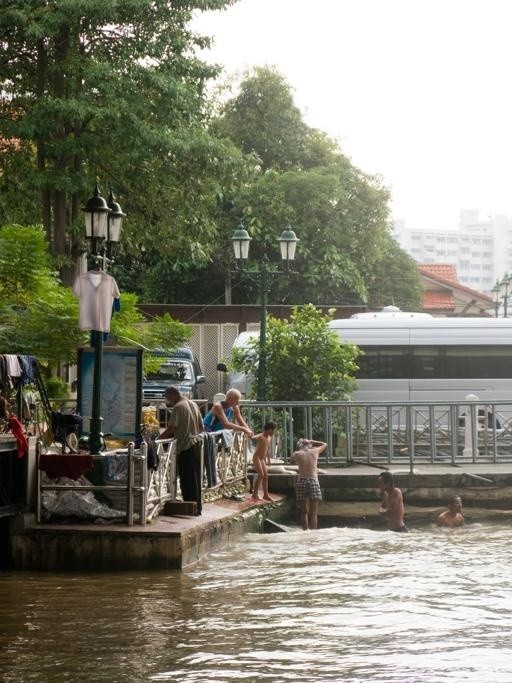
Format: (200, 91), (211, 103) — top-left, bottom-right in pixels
(88, 260), (103, 280)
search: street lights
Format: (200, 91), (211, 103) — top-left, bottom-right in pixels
(491, 272), (512, 318)
(81, 179), (128, 455)
(230, 217), (299, 402)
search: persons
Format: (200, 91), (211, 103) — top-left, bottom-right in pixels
(289, 437), (328, 528)
(436, 493), (466, 527)
(202, 388), (255, 436)
(157, 385), (206, 515)
(249, 420), (276, 501)
(375, 469), (407, 532)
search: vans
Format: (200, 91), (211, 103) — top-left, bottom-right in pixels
(141, 348), (205, 400)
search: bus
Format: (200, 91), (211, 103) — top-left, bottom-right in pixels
(216, 312), (512, 430)
